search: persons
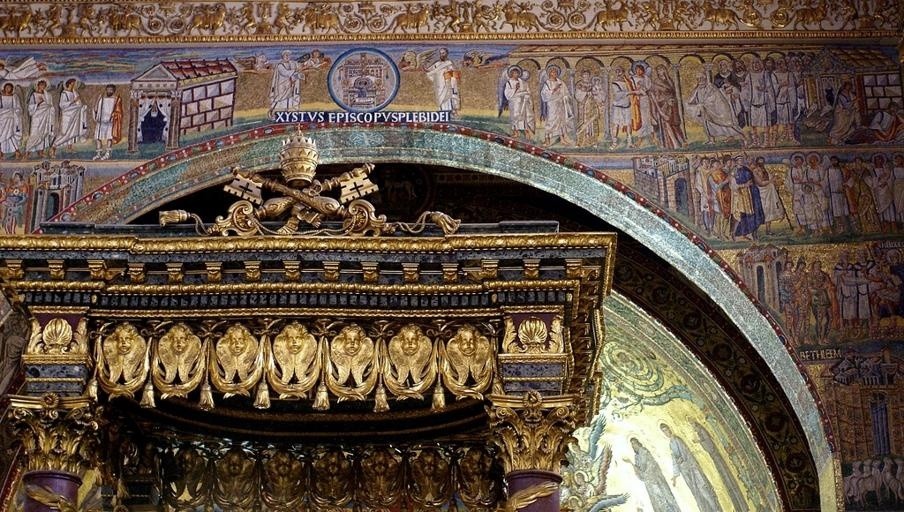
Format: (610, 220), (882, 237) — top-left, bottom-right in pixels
(103, 323), (147, 384)
(166, 441), (496, 505)
(329, 322), (374, 388)
(273, 320), (318, 385)
(157, 321), (201, 381)
(388, 322), (432, 385)
(216, 322), (259, 384)
(447, 322), (490, 387)
